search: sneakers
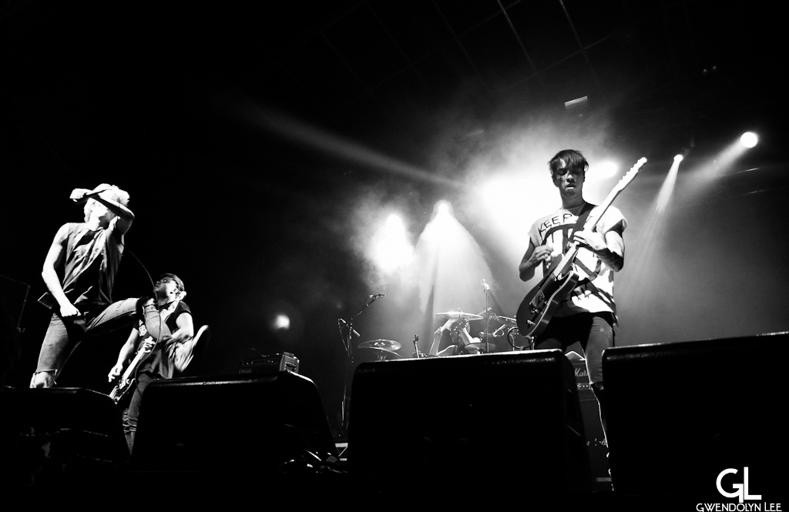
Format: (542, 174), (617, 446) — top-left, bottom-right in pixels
(172, 324), (212, 374)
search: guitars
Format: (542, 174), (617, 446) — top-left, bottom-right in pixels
(516, 156), (648, 339)
(102, 335), (158, 418)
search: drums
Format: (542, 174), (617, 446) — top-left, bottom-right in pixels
(345, 346), (403, 400)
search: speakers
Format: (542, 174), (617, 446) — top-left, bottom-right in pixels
(0, 331), (789, 496)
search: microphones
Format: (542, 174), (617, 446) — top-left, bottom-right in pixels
(76, 311), (92, 319)
(340, 318), (361, 338)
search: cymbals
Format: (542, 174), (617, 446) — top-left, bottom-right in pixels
(466, 342), (494, 351)
(357, 339), (402, 352)
(435, 309), (482, 321)
(488, 315), (517, 328)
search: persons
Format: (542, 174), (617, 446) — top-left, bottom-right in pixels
(429, 312), (485, 358)
(104, 271), (196, 455)
(517, 147), (628, 495)
(28, 182), (209, 389)
